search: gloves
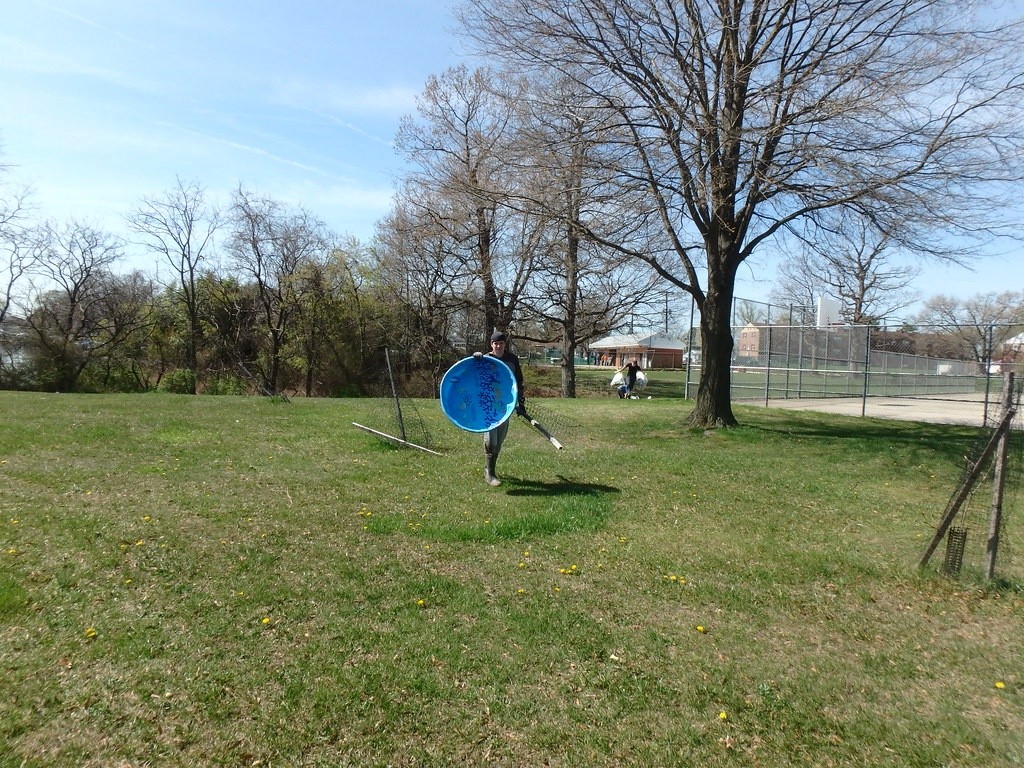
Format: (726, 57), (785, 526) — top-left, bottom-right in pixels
(516, 404), (526, 417)
(473, 351), (483, 362)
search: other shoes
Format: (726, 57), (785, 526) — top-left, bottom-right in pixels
(484, 468), (502, 486)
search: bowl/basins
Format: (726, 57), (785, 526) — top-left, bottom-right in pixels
(440, 356), (519, 434)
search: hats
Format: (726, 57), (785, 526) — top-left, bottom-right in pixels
(491, 332), (506, 342)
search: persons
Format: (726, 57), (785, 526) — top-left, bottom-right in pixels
(473, 330), (526, 487)
(591, 353), (642, 399)
